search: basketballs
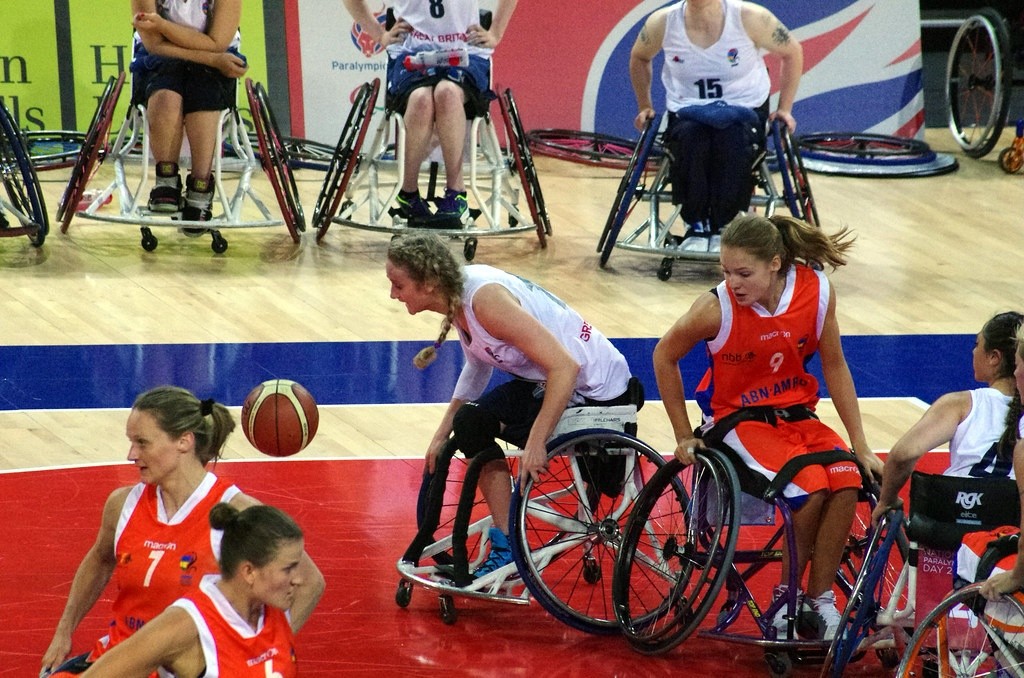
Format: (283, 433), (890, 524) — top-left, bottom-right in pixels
(240, 378), (318, 457)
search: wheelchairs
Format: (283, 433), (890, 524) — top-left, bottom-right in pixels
(55, 68), (307, 254)
(609, 440), (1023, 677)
(311, 7), (553, 262)
(941, 0), (1024, 176)
(393, 374), (696, 637)
(0, 103), (48, 245)
(596, 93), (820, 282)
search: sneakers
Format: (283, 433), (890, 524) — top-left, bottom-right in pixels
(396, 188), (434, 224)
(799, 589), (847, 641)
(181, 172), (215, 238)
(149, 160), (183, 212)
(707, 232), (722, 253)
(768, 584), (803, 641)
(471, 527), (521, 580)
(435, 187), (469, 224)
(681, 236), (709, 252)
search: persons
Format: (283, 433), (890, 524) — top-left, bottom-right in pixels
(38, 383), (328, 678)
(383, 232), (640, 581)
(342, 0), (520, 228)
(648, 213), (888, 641)
(80, 500), (305, 677)
(628, 0), (804, 254)
(870, 309), (1024, 678)
(128, 0), (251, 238)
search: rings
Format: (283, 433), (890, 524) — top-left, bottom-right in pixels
(687, 447), (695, 454)
